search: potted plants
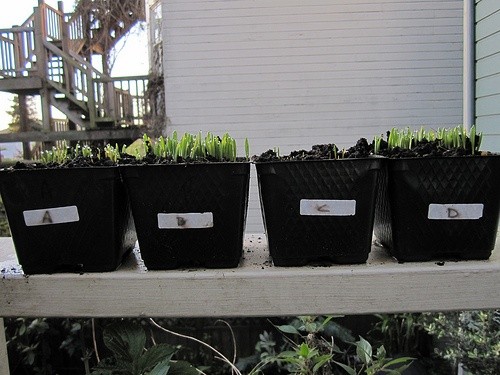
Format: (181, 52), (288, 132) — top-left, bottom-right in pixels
(0, 139), (139, 276)
(126, 131), (250, 272)
(250, 139), (388, 268)
(370, 123), (500, 263)
